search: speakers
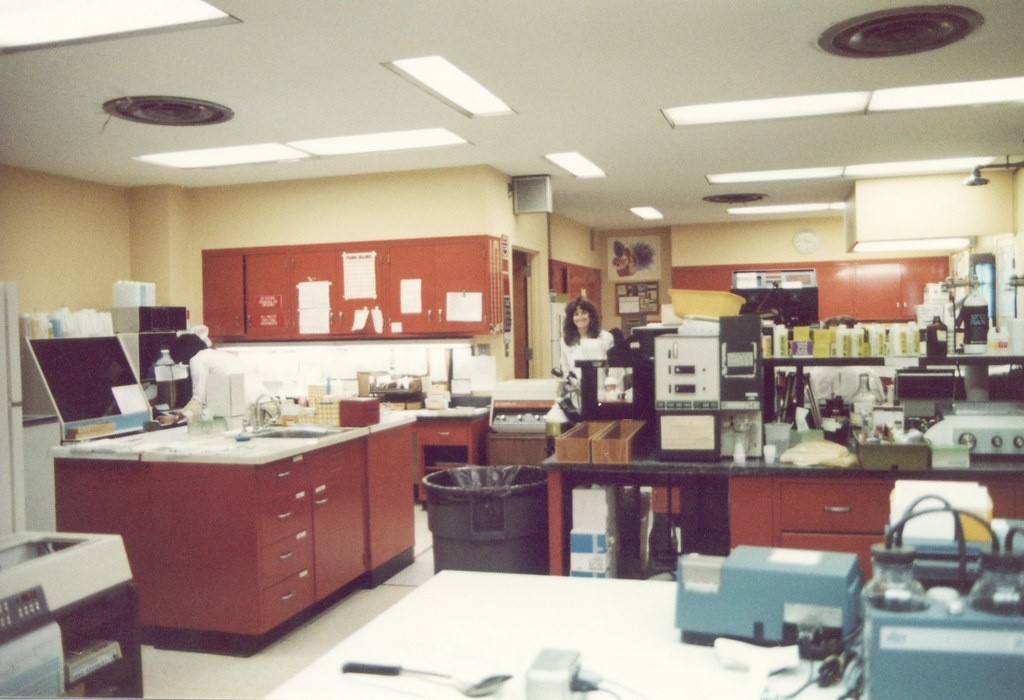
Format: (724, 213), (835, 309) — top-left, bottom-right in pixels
(513, 175), (552, 214)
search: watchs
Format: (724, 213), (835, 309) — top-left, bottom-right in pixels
(174, 414), (178, 422)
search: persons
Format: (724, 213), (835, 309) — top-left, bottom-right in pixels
(609, 328), (633, 392)
(562, 297), (627, 411)
(155, 334), (253, 426)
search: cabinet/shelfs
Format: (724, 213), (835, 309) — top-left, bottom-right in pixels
(259, 440), (372, 634)
(202, 250), (293, 343)
(776, 475), (897, 584)
(417, 419), (486, 508)
(292, 246), (388, 339)
(389, 240), (489, 335)
(853, 258), (946, 320)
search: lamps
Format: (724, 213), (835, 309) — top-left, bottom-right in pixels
(963, 159), (1024, 185)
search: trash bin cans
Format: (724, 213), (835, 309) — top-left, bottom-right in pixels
(423, 464), (548, 576)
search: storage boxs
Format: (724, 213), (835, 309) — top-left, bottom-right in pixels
(569, 484), (623, 578)
(555, 419), (617, 465)
(591, 416), (650, 464)
